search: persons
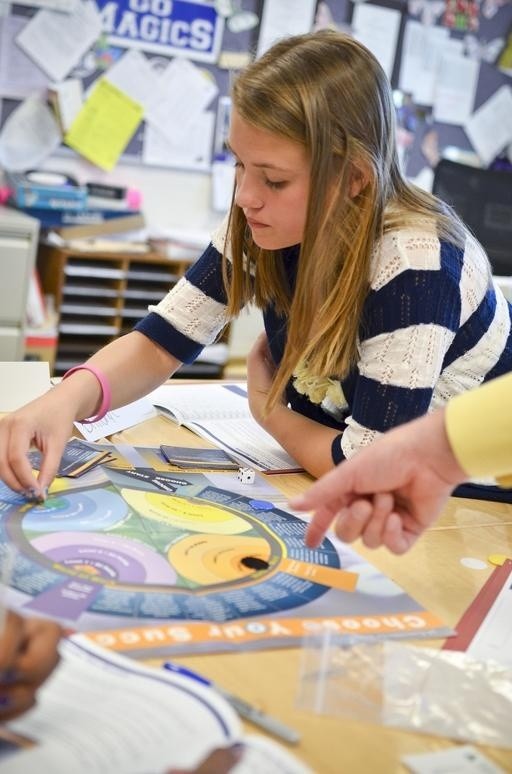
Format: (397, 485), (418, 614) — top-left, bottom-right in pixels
(0, 608), (245, 774)
(288, 372), (511, 559)
(2, 27), (511, 501)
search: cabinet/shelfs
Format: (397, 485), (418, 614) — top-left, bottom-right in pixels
(1, 203), (42, 361)
(38, 236), (236, 378)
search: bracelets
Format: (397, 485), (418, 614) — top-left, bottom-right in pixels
(62, 363), (111, 424)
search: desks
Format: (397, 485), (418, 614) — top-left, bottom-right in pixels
(3, 378), (512, 771)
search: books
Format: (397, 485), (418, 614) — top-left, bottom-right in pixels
(151, 384), (304, 474)
(0, 634), (307, 774)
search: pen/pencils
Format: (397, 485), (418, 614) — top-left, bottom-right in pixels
(165, 663), (299, 743)
